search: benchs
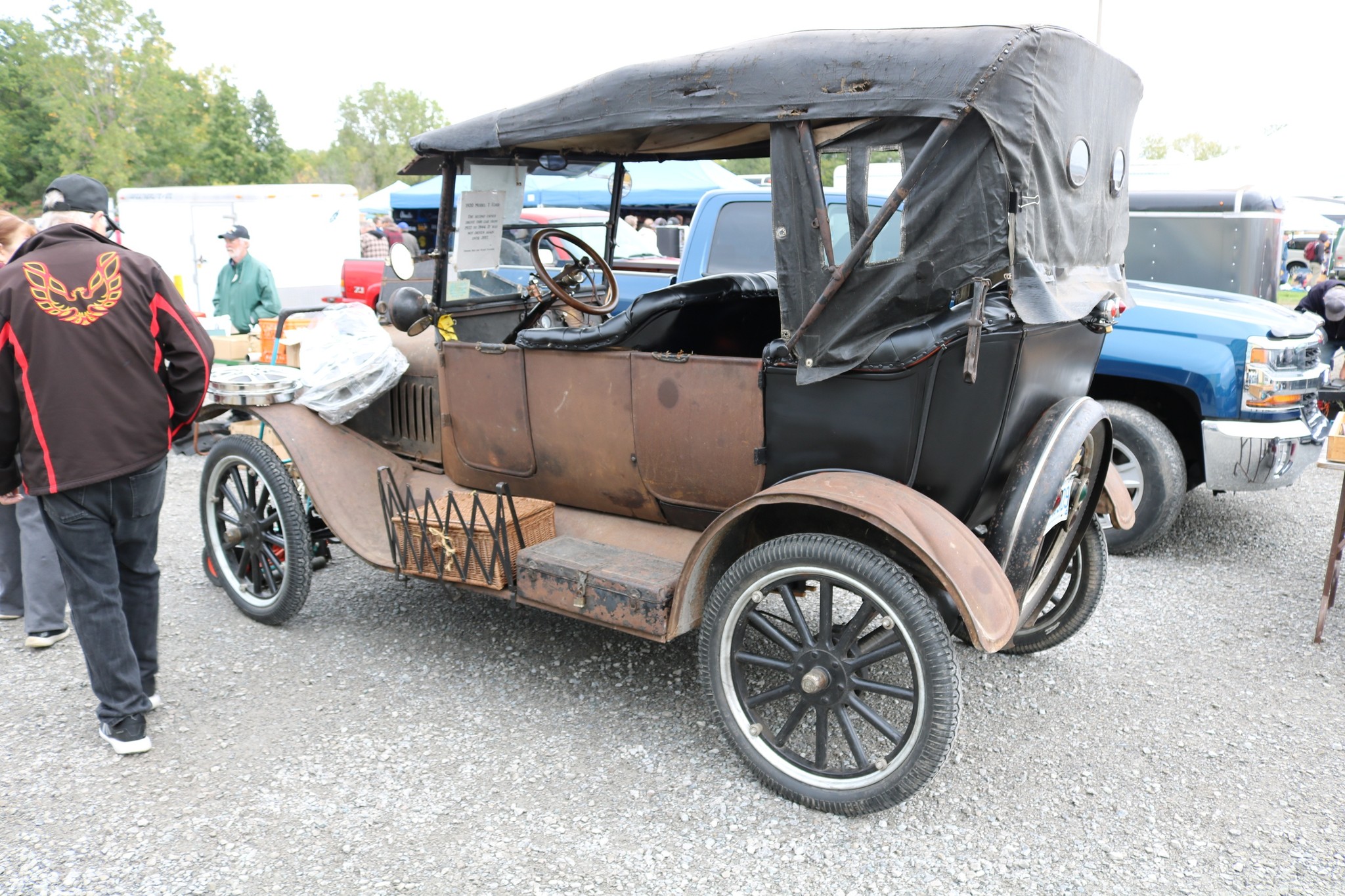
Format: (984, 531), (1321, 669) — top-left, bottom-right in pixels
(517, 270), (780, 359)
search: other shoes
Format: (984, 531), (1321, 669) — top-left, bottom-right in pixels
(227, 414), (252, 422)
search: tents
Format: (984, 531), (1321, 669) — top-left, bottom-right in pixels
(356, 179), (413, 217)
(392, 170), (768, 267)
(588, 155), (736, 188)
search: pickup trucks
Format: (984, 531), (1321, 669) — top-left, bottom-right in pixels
(466, 187), (1335, 560)
(320, 202), (684, 319)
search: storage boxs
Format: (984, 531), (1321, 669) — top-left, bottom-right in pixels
(256, 318), (314, 364)
(208, 333), (258, 361)
(227, 421), (292, 470)
(391, 490), (554, 590)
(1326, 411), (1345, 462)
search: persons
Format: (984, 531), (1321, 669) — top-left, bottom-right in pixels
(212, 225), (281, 427)
(1293, 276), (1345, 442)
(1302, 232), (1328, 289)
(622, 208), (699, 257)
(0, 171), (214, 755)
(355, 211), (435, 269)
(0, 208), (73, 649)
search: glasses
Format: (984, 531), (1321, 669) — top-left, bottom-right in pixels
(105, 219), (116, 238)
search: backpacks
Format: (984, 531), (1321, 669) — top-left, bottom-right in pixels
(1304, 240), (1324, 260)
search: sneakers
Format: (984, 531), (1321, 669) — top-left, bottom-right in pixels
(99, 714), (152, 755)
(24, 621), (71, 648)
(141, 685), (161, 711)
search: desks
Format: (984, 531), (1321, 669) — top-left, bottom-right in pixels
(1314, 436), (1345, 644)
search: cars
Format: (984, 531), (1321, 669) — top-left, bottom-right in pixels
(1281, 233), (1336, 279)
(198, 27), (1137, 818)
(1331, 225), (1345, 280)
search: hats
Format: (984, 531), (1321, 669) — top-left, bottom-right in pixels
(649, 217), (667, 227)
(397, 222), (409, 229)
(1319, 230), (1328, 242)
(42, 174), (124, 233)
(381, 216), (394, 223)
(667, 217), (680, 225)
(218, 225), (250, 240)
(1323, 288), (1345, 321)
